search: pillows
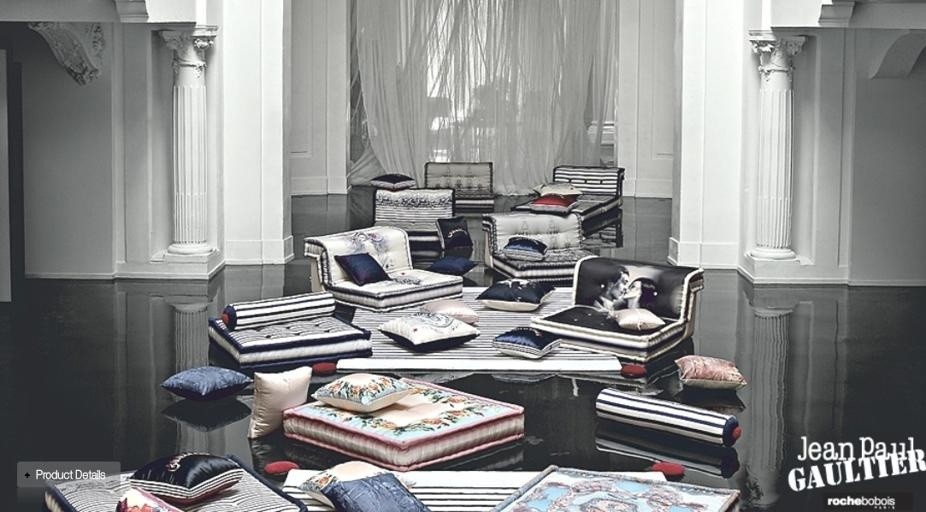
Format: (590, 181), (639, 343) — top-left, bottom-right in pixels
(594, 351), (749, 446)
(221, 290), (335, 331)
(116, 360), (428, 512)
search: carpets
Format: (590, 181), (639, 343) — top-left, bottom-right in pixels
(278, 463), (667, 512)
(336, 284), (624, 375)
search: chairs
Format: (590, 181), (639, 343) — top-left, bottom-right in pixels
(303, 161), (705, 363)
